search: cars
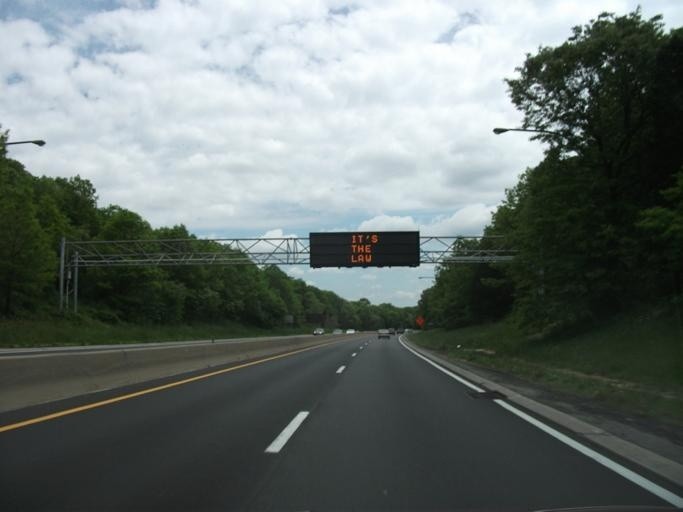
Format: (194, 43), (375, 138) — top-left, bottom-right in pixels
(313, 328), (360, 336)
(378, 326), (404, 339)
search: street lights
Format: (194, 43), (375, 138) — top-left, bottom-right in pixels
(492, 126), (582, 154)
(0, 139), (46, 150)
